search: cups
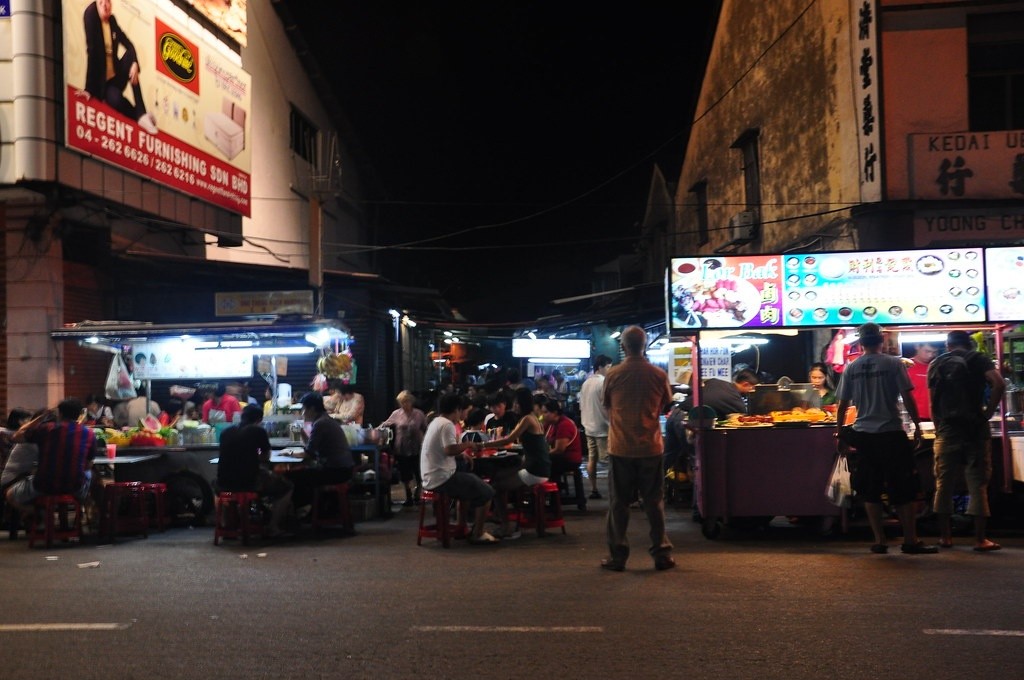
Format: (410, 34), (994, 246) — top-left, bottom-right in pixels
(293, 427), (301, 441)
(105, 444), (116, 459)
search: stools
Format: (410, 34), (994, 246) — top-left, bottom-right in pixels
(516, 481), (566, 537)
(417, 491), (457, 549)
(142, 483), (169, 532)
(213, 493), (266, 546)
(103, 482), (151, 539)
(28, 493), (87, 549)
(313, 483), (356, 535)
(554, 468), (587, 513)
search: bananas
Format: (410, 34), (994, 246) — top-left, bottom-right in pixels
(318, 353), (353, 376)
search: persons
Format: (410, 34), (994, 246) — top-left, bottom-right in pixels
(807, 362), (840, 405)
(578, 352), (610, 500)
(416, 367), (582, 543)
(906, 339), (941, 421)
(223, 379), (282, 417)
(285, 392), (355, 534)
(321, 378), (366, 427)
(658, 400), (679, 438)
(75, 0), (161, 134)
(378, 390), (429, 505)
(837, 322), (940, 554)
(0, 392), (115, 546)
(113, 388), (202, 429)
(215, 402), (293, 532)
(926, 330), (1005, 551)
(201, 382), (241, 421)
(661, 368), (761, 510)
(598, 326), (677, 571)
(982, 361), (1016, 410)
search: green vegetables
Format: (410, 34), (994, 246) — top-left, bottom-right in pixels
(95, 432), (113, 439)
(160, 427), (172, 438)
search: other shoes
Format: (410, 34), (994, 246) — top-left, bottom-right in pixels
(655, 557), (674, 569)
(902, 540), (937, 553)
(600, 559), (624, 571)
(590, 491), (600, 499)
(871, 544), (887, 554)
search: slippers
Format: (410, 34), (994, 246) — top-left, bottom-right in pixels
(454, 528), (471, 539)
(937, 538), (952, 547)
(470, 533), (501, 545)
(974, 539), (1001, 551)
(491, 528), (512, 538)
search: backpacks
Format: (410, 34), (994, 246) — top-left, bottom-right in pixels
(934, 349), (983, 425)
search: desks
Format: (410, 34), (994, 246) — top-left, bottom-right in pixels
(209, 450), (304, 475)
(92, 452), (161, 464)
(468, 451), (522, 472)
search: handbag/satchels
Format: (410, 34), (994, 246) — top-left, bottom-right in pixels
(104, 355), (137, 400)
(828, 456), (864, 519)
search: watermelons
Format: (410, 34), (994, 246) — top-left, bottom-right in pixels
(138, 413), (162, 433)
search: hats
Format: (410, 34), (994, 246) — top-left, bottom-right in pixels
(859, 323), (882, 345)
(948, 331), (969, 345)
